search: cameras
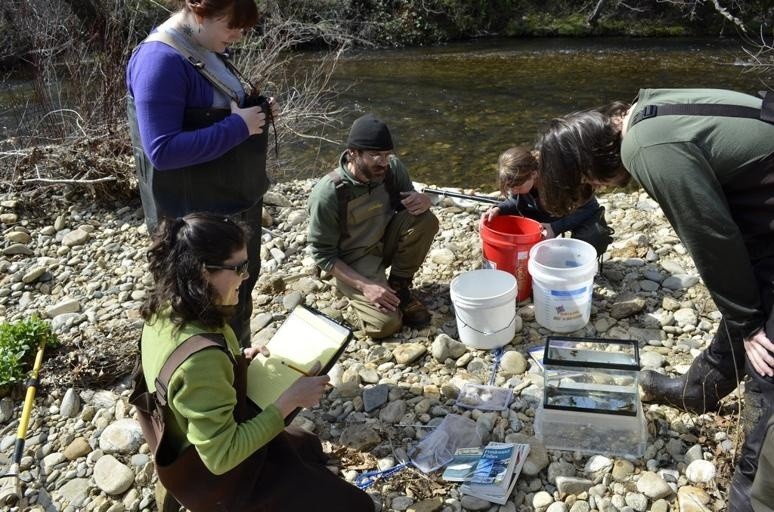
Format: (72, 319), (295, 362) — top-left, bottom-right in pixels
(237, 93), (271, 126)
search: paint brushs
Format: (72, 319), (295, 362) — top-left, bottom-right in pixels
(281, 361), (337, 389)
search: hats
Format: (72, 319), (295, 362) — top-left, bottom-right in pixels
(346, 115), (395, 152)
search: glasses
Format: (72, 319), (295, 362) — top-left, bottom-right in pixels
(366, 153), (396, 163)
(205, 259), (249, 276)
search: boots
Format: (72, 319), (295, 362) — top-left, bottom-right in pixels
(638, 315), (747, 416)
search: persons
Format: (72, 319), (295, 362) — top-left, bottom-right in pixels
(125, 206), (377, 512)
(125, 0), (284, 356)
(305, 113), (444, 342)
(475, 142), (616, 259)
(532, 83), (773, 511)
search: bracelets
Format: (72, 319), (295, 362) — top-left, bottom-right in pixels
(238, 345), (249, 360)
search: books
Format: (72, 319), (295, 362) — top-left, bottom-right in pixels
(458, 440), (532, 507)
(238, 302), (353, 429)
(469, 443), (520, 498)
(441, 443), (485, 483)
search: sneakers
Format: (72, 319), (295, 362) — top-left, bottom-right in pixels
(154, 473), (182, 511)
(388, 280), (432, 330)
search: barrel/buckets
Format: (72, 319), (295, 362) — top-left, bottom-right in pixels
(527, 237), (599, 333)
(449, 270), (518, 350)
(480, 214), (543, 303)
